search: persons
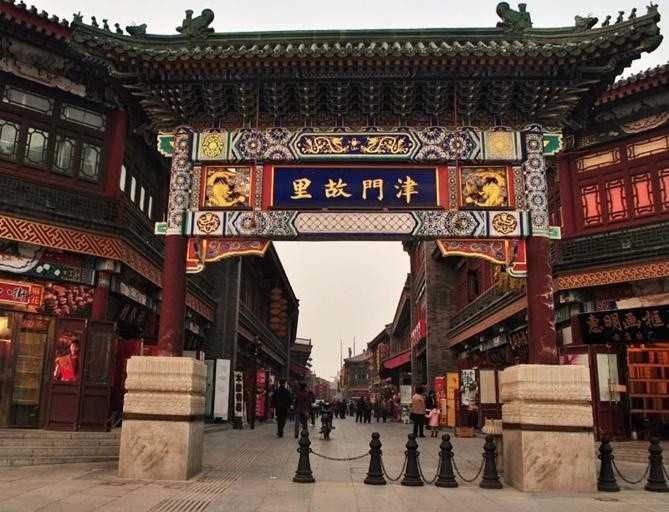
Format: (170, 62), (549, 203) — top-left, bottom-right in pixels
(269, 379), (439, 439)
(54, 339), (81, 381)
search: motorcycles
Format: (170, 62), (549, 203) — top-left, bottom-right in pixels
(316, 400), (337, 441)
